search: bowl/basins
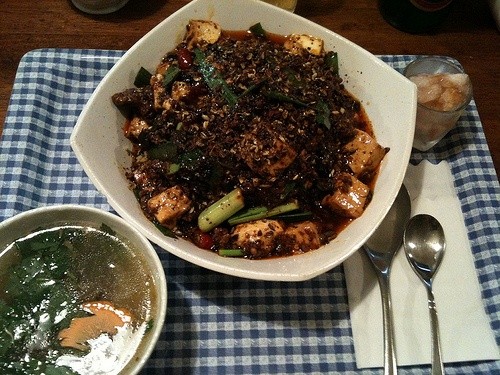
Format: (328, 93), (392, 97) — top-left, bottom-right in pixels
(0, 204), (168, 375)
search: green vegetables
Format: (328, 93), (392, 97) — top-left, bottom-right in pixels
(0, 230), (155, 375)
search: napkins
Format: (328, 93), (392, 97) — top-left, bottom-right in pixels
(344, 159), (500, 369)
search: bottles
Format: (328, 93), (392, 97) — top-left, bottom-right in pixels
(378, 0), (455, 34)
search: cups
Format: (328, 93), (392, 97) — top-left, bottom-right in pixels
(403, 57), (473, 152)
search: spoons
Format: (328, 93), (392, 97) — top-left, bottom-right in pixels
(404, 214), (446, 375)
(362, 181), (411, 375)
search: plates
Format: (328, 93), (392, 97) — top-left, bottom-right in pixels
(69, 0), (417, 281)
